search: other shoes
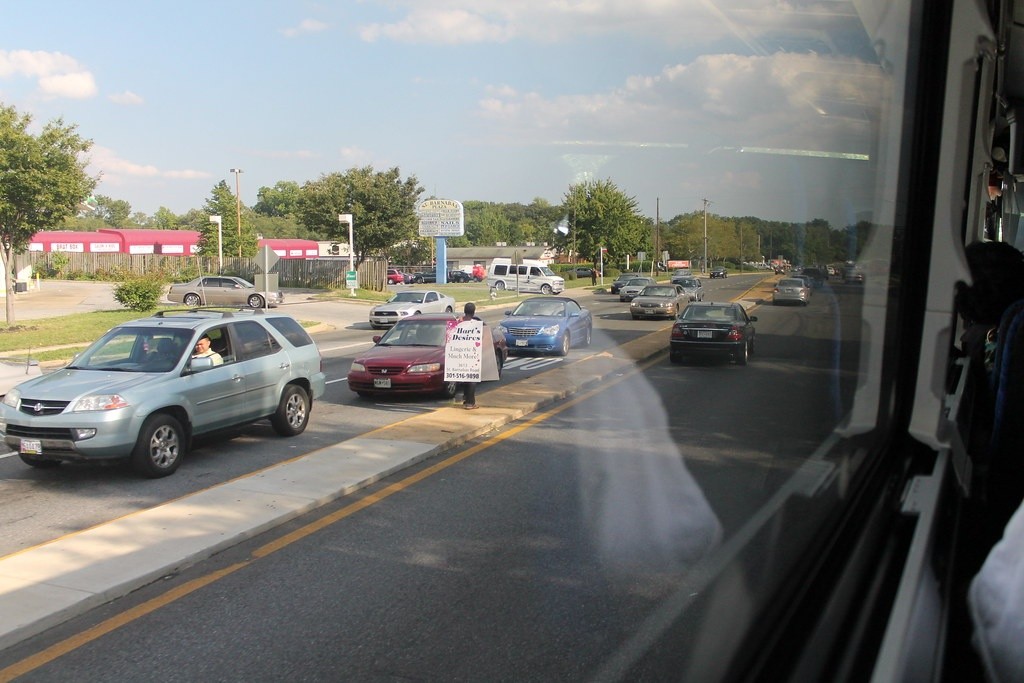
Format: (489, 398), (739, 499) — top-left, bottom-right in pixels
(463, 404), (479, 409)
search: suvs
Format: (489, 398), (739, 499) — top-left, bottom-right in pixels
(673, 277), (704, 303)
(0, 306), (324, 478)
(387, 268), (404, 284)
(671, 269), (694, 283)
(416, 266), (454, 284)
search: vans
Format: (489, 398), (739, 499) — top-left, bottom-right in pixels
(461, 266), (485, 282)
(485, 258), (565, 296)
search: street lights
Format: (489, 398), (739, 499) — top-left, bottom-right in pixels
(210, 215), (222, 275)
(229, 168), (245, 257)
(339, 214), (355, 297)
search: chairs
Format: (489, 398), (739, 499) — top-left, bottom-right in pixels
(149, 338), (175, 364)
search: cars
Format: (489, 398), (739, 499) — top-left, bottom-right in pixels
(167, 275), (285, 309)
(629, 283), (690, 319)
(452, 270), (472, 283)
(403, 272), (416, 284)
(369, 290), (455, 329)
(773, 278), (811, 306)
(670, 302), (759, 365)
(347, 313), (510, 398)
(497, 296), (592, 353)
(619, 277), (657, 302)
(567, 267), (601, 277)
(709, 267), (729, 278)
(611, 273), (641, 294)
(776, 264), (866, 296)
(755, 260), (791, 270)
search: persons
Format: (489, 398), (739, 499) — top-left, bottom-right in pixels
(452, 302), (486, 410)
(185, 333), (224, 370)
(591, 267), (597, 286)
(954, 238), (1024, 683)
